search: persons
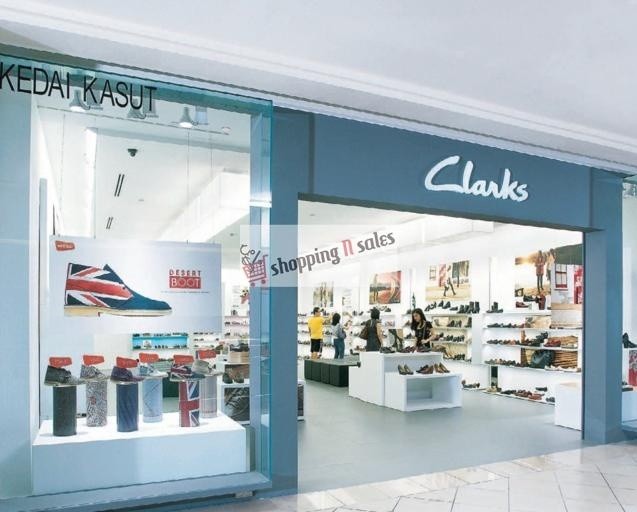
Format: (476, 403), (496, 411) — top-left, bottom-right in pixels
(331, 312), (351, 359)
(312, 280), (329, 307)
(410, 308), (436, 352)
(442, 265), (456, 296)
(307, 307), (326, 360)
(364, 308), (384, 350)
(533, 248), (557, 294)
(372, 274), (378, 303)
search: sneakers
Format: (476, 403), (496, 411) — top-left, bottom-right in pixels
(45, 360), (244, 386)
(225, 385), (303, 421)
(64, 263), (172, 317)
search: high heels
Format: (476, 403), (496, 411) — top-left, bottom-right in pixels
(398, 363), (448, 375)
(380, 345), (431, 353)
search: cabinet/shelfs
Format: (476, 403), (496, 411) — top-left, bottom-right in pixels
(131, 333), (188, 349)
(225, 310), (585, 427)
(192, 330), (223, 361)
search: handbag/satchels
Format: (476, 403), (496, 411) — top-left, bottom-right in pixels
(530, 351), (554, 368)
(359, 327), (368, 339)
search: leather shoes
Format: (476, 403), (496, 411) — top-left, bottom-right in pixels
(425, 301), (479, 360)
(231, 345), (248, 352)
(516, 295), (545, 309)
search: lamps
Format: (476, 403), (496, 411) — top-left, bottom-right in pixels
(69, 87), (196, 130)
